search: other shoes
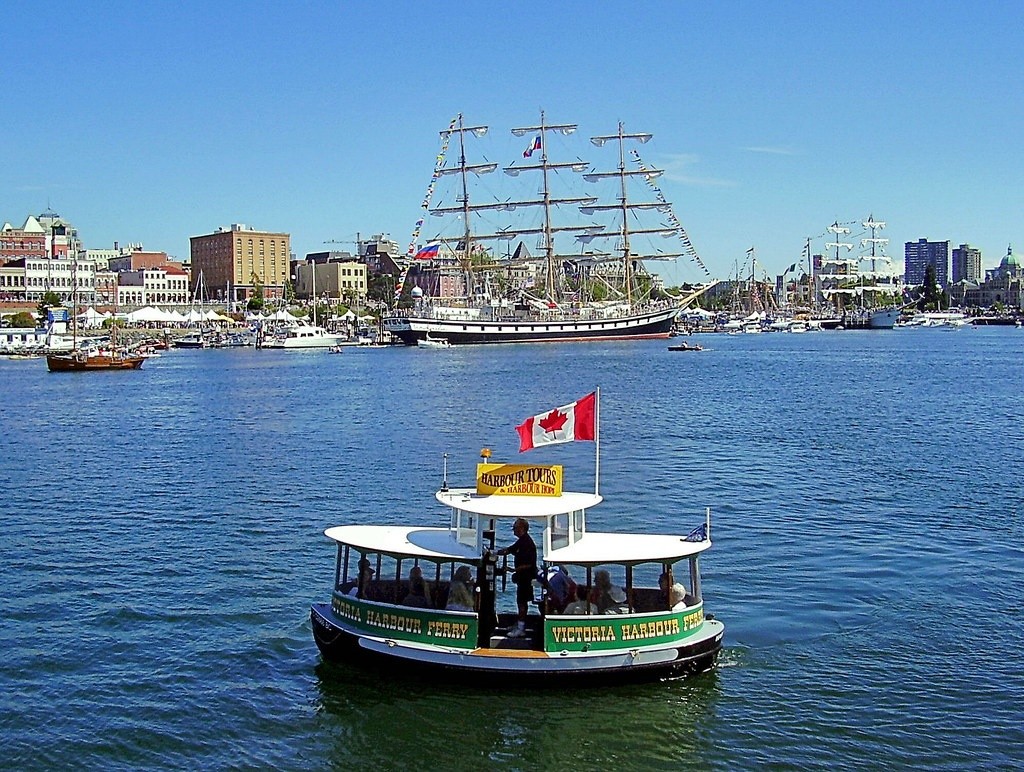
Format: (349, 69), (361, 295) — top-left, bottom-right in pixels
(507, 627), (527, 638)
(507, 623), (518, 631)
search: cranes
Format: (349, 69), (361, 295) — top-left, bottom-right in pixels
(323, 232), (370, 254)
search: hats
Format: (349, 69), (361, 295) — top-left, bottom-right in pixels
(607, 586), (627, 603)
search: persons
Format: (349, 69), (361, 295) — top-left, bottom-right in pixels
(681, 340), (700, 348)
(350, 559), (475, 612)
(537, 563), (686, 615)
(80, 340), (88, 361)
(489, 519), (537, 637)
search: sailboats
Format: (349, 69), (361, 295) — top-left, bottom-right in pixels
(172, 259), (345, 346)
(374, 108), (726, 341)
(32, 240), (161, 370)
(731, 214), (925, 329)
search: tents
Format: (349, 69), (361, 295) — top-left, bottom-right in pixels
(743, 311), (766, 320)
(681, 307), (715, 317)
(124, 306), (221, 323)
(327, 308), (375, 325)
(73, 307), (105, 330)
(246, 308), (312, 322)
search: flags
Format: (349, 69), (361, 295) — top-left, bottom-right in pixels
(414, 239), (443, 260)
(523, 133), (542, 158)
(515, 391), (596, 452)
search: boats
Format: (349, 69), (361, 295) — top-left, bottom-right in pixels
(895, 313), (973, 326)
(312, 448), (726, 693)
(135, 336), (167, 349)
(668, 344), (708, 350)
(417, 337), (454, 349)
(672, 320), (807, 336)
